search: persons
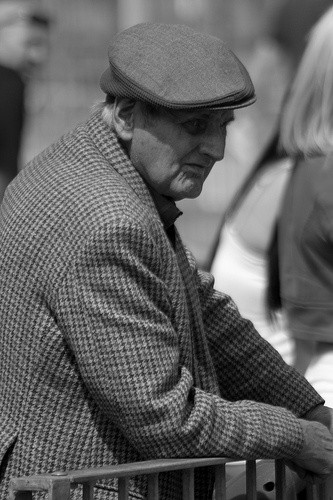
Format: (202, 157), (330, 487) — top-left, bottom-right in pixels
(0, 7), (49, 202)
(0, 21), (333, 500)
(206, 5), (333, 411)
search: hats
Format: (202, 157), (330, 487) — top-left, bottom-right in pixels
(99, 23), (256, 113)
